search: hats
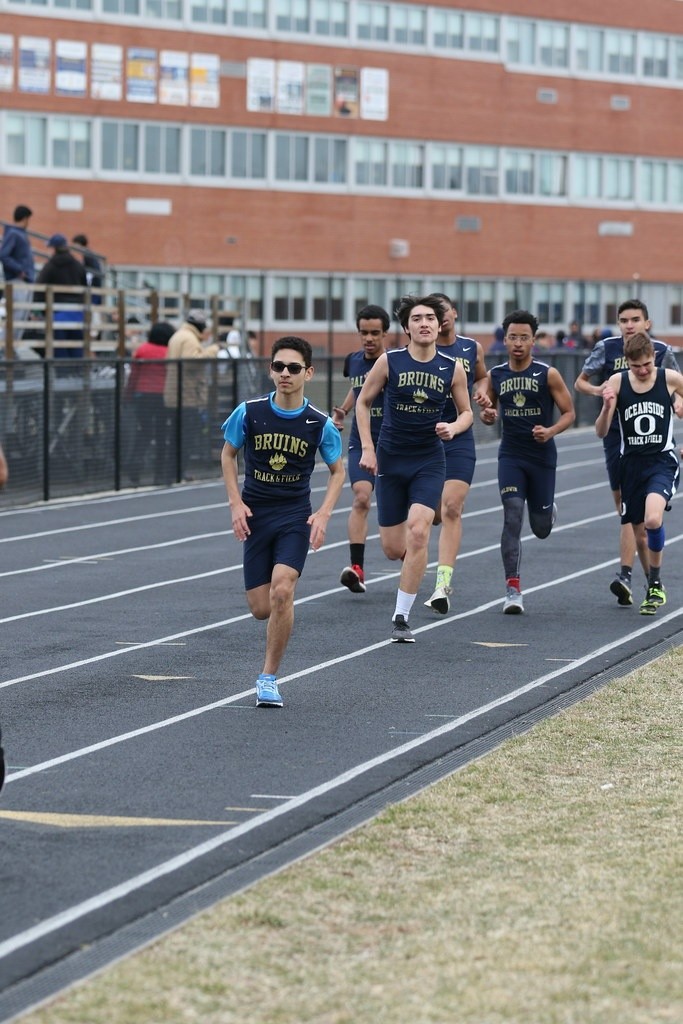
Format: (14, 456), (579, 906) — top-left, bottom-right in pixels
(226, 330), (242, 345)
(44, 234), (67, 248)
(187, 308), (208, 324)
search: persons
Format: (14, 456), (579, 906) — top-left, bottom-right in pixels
(332, 304), (390, 592)
(490, 321), (612, 348)
(355, 298), (473, 643)
(480, 311), (576, 612)
(0, 205), (100, 377)
(123, 311), (256, 485)
(574, 299), (683, 605)
(424, 293), (492, 614)
(594, 332), (683, 615)
(220, 337), (345, 707)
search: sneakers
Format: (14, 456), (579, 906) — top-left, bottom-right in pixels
(340, 562), (367, 593)
(390, 614), (416, 642)
(423, 586), (454, 615)
(503, 585), (524, 614)
(640, 599), (657, 615)
(643, 581), (666, 606)
(254, 674), (283, 707)
(550, 502), (557, 525)
(609, 573), (633, 606)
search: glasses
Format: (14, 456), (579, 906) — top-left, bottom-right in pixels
(270, 362), (308, 374)
(505, 336), (534, 343)
